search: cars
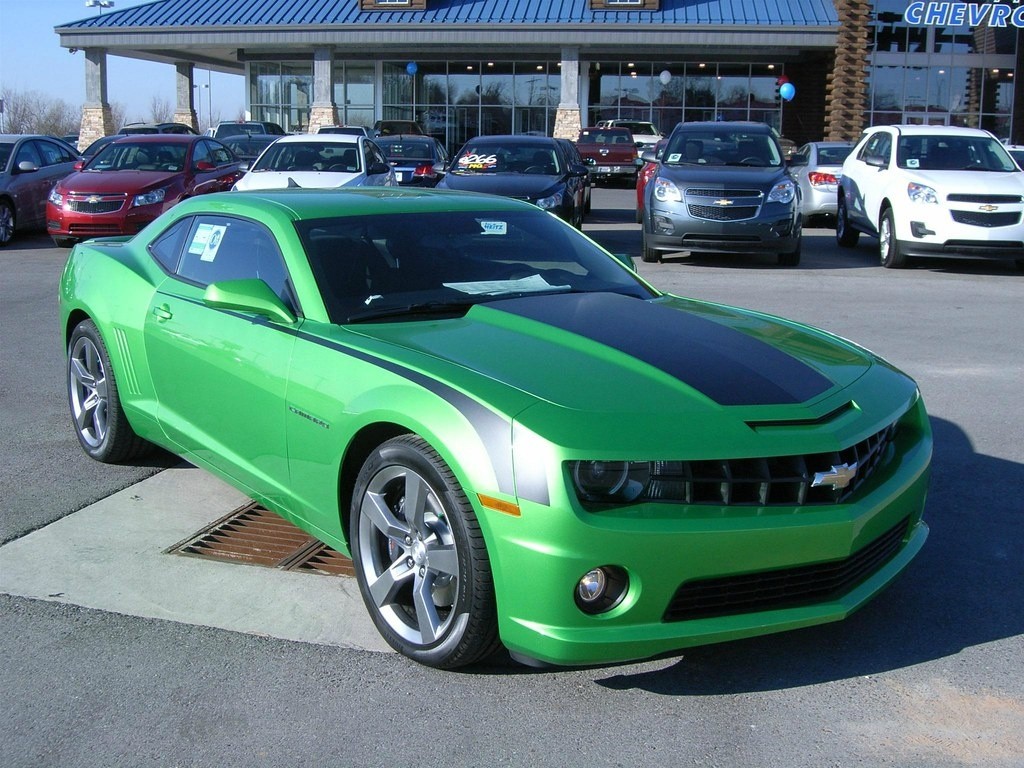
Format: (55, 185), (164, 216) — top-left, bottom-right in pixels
(551, 138), (592, 221)
(214, 133), (296, 179)
(229, 134), (398, 192)
(786, 141), (859, 227)
(77, 128), (185, 178)
(202, 128), (219, 139)
(607, 120), (665, 160)
(426, 113), (509, 135)
(769, 124), (797, 161)
(572, 128), (644, 186)
(636, 139), (740, 224)
(998, 138), (1010, 145)
(595, 120), (616, 131)
(369, 135), (451, 190)
(0, 133), (87, 247)
(521, 131), (547, 137)
(431, 135), (589, 237)
(1003, 144), (1024, 175)
(46, 134), (248, 248)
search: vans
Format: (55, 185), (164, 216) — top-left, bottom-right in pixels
(372, 120), (425, 137)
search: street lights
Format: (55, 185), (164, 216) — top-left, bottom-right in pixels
(191, 84), (208, 136)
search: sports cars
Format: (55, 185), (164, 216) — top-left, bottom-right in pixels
(57, 186), (935, 672)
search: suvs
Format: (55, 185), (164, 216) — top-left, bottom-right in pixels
(211, 121), (296, 150)
(116, 122), (208, 162)
(641, 121), (808, 266)
(834, 124), (1024, 277)
(316, 124), (376, 144)
